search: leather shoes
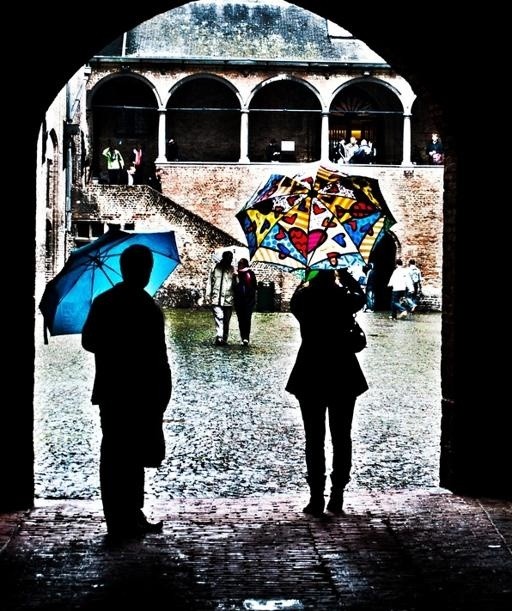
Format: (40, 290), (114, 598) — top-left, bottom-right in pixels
(145, 517), (165, 534)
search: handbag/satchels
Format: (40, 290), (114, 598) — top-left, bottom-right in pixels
(351, 314), (368, 354)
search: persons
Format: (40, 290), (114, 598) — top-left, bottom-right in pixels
(285, 269), (369, 514)
(426, 128), (444, 164)
(329, 136), (393, 164)
(405, 259), (422, 313)
(82, 245), (172, 538)
(386, 259), (416, 320)
(102, 138), (186, 186)
(363, 263), (378, 313)
(205, 251), (256, 346)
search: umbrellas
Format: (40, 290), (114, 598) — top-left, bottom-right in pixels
(39, 228), (183, 345)
(235, 163), (397, 273)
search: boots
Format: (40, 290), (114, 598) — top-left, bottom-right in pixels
(304, 474), (352, 516)
